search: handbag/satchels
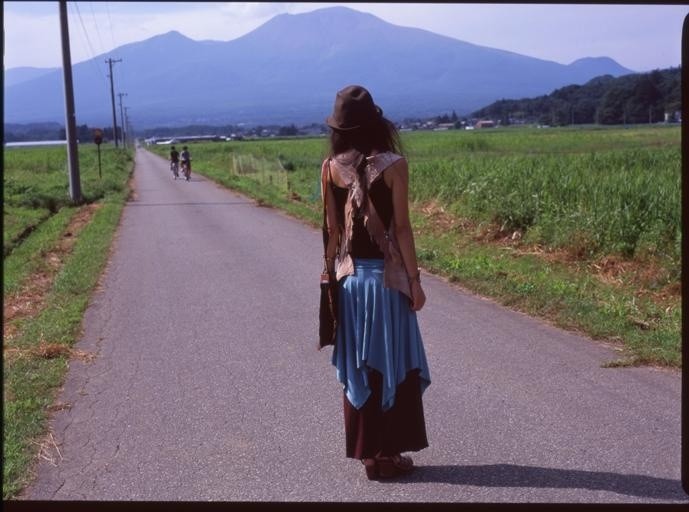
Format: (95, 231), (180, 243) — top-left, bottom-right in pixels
(318, 268), (340, 349)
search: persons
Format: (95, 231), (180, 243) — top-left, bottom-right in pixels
(180, 146), (192, 180)
(168, 146), (179, 177)
(316, 85), (431, 479)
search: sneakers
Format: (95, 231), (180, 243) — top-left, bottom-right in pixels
(365, 452), (414, 481)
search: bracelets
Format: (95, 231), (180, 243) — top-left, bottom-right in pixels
(408, 270), (419, 283)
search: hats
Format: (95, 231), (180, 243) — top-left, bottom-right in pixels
(326, 84), (381, 131)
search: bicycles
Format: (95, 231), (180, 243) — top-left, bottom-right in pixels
(169, 158), (193, 181)
